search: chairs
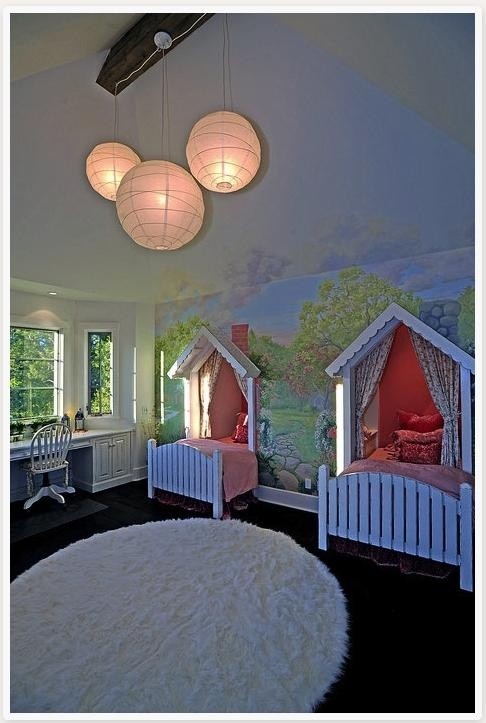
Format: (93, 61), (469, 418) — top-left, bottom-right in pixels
(20, 423), (76, 510)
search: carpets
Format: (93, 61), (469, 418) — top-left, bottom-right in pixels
(10, 493), (109, 542)
(10, 518), (351, 713)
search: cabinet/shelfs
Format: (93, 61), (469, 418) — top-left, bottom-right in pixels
(70, 432), (131, 493)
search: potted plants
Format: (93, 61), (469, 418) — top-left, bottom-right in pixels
(10, 418), (58, 442)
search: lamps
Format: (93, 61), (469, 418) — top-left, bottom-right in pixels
(84, 13), (261, 252)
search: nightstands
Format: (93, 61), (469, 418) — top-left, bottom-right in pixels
(363, 429), (378, 459)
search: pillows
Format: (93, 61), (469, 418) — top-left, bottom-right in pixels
(388, 428), (444, 444)
(235, 425), (248, 443)
(395, 408), (444, 433)
(387, 441), (441, 465)
(231, 411), (248, 439)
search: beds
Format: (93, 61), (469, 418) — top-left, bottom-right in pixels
(318, 302), (475, 593)
(147, 325), (259, 518)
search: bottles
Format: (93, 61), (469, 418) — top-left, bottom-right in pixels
(59, 413), (71, 434)
(74, 408), (85, 430)
